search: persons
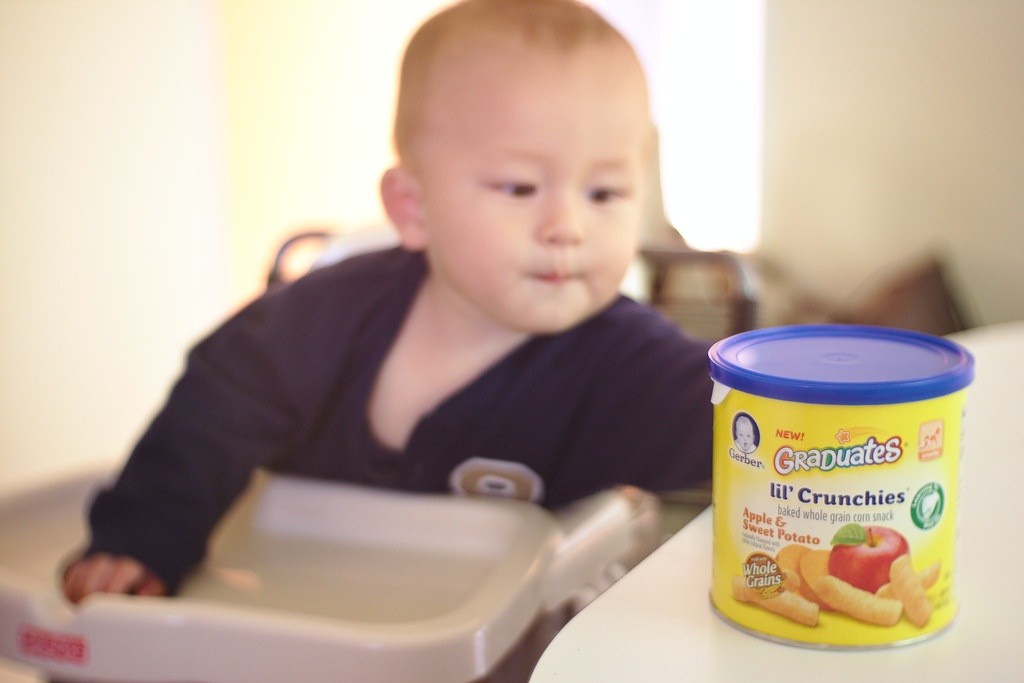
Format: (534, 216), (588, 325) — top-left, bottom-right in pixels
(64, 1), (713, 596)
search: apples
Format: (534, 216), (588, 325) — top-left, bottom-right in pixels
(827, 526), (909, 593)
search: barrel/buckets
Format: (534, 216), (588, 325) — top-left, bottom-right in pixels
(705, 325), (977, 649)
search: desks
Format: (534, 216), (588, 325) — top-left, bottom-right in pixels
(526, 320), (1024, 683)
